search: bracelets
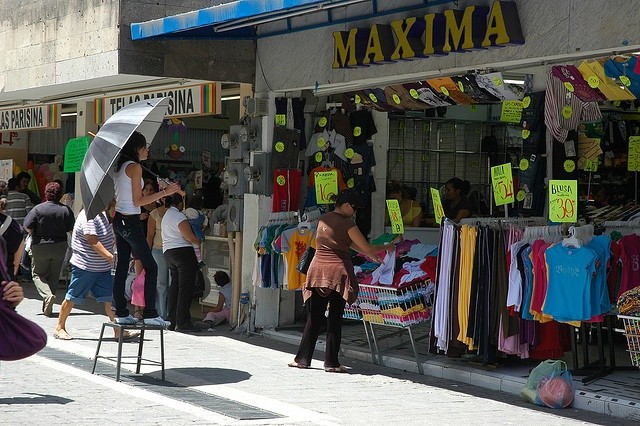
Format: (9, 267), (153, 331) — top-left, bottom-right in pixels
(163, 190), (167, 196)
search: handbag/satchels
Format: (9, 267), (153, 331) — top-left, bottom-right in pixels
(0, 253), (46, 360)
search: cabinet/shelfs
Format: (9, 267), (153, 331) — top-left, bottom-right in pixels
(198, 236), (235, 308)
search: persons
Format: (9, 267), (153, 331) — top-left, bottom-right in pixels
(161, 192), (204, 332)
(6, 178), (35, 271)
(182, 194), (208, 271)
(0, 181), (25, 282)
(53, 206), (142, 340)
(146, 195), (172, 323)
(384, 184), (423, 227)
(0, 234), (24, 314)
(424, 177), (472, 226)
(194, 271), (233, 330)
(23, 180), (75, 318)
(58, 192), (74, 281)
(288, 188), (395, 373)
(17, 172), (42, 207)
(113, 131), (171, 327)
(139, 182), (161, 237)
(131, 259), (147, 325)
(517, 150), (536, 194)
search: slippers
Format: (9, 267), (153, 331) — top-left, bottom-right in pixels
(54, 330), (73, 340)
(114, 330), (140, 341)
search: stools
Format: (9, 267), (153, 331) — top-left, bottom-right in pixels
(89, 322), (167, 381)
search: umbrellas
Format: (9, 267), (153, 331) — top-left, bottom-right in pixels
(74, 93), (187, 220)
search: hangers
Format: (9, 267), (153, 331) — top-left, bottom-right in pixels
(330, 100), (337, 114)
(603, 220), (640, 236)
(324, 120), (334, 133)
(299, 210), (322, 228)
(443, 215), (543, 228)
(267, 210), (299, 225)
(322, 141), (335, 153)
(354, 99), (364, 111)
(560, 223), (596, 247)
(321, 152), (334, 170)
(517, 223), (566, 242)
(611, 53), (634, 62)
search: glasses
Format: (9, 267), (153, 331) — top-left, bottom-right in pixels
(349, 202), (357, 211)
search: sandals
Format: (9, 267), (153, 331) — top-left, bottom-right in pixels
(325, 365), (348, 373)
(287, 361), (308, 369)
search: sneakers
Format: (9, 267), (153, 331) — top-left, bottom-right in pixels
(144, 317), (171, 326)
(175, 323), (203, 331)
(43, 295), (56, 316)
(194, 319), (214, 329)
(114, 313), (138, 324)
